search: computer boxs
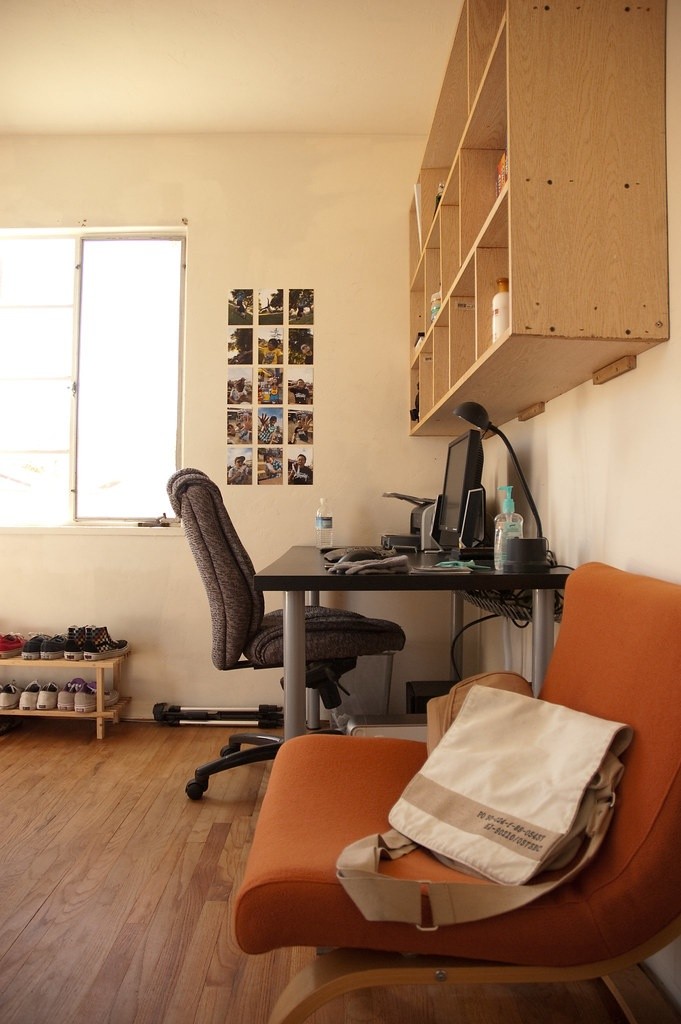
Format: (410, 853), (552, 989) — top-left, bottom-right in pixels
(405, 680), (457, 714)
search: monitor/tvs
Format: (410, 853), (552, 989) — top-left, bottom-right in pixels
(440, 429), (494, 562)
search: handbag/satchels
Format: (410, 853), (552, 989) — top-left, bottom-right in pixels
(426, 671), (536, 758)
(336, 683), (633, 932)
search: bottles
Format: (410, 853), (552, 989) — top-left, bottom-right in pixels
(316, 498), (333, 548)
(492, 277), (509, 342)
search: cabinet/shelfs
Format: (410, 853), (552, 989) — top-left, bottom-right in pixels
(0, 649), (131, 738)
(408, 0), (667, 438)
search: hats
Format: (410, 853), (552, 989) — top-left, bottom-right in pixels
(235, 456), (245, 463)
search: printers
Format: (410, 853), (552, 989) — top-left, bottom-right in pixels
(381, 496), (443, 555)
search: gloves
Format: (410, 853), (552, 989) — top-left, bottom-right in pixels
(328, 554), (411, 575)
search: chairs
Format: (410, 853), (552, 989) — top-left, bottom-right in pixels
(165, 466), (407, 799)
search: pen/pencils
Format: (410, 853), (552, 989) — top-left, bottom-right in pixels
(424, 550), (438, 554)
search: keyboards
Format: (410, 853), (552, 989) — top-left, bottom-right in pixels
(324, 547), (397, 563)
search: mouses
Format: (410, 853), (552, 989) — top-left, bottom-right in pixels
(337, 550), (381, 564)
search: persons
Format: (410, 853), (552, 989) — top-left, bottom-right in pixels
(227, 293), (314, 485)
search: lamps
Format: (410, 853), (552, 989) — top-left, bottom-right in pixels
(454, 401), (553, 573)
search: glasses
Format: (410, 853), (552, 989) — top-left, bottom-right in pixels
(237, 461), (243, 463)
(236, 422), (241, 426)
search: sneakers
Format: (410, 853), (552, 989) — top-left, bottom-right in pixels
(84, 626), (130, 660)
(0, 683), (23, 709)
(64, 625), (87, 661)
(40, 633), (68, 660)
(21, 632), (44, 660)
(74, 682), (120, 713)
(36, 681), (60, 709)
(57, 678), (87, 710)
(0, 633), (26, 659)
(18, 681), (41, 710)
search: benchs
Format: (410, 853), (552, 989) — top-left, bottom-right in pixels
(235, 562), (681, 1024)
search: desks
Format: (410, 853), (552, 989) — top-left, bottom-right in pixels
(253, 546), (576, 744)
